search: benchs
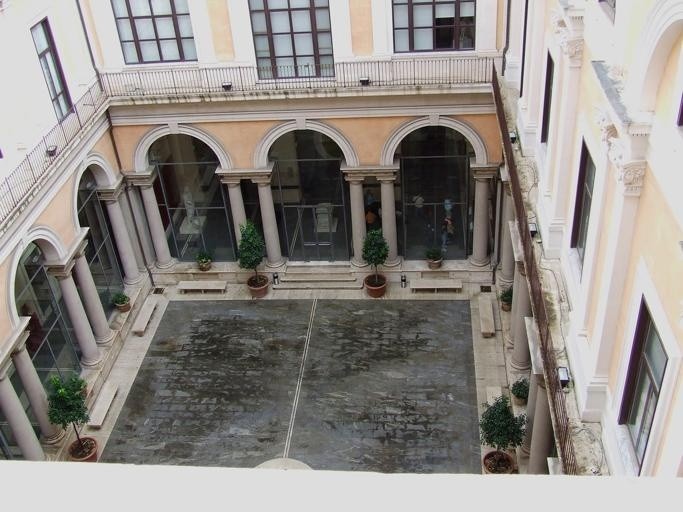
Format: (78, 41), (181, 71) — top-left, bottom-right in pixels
(87, 383), (119, 430)
(486, 385), (501, 408)
(131, 294), (159, 336)
(410, 279), (462, 293)
(177, 280), (227, 294)
(478, 295), (496, 337)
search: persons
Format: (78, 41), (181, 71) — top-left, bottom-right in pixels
(363, 189), (375, 206)
(411, 190), (456, 259)
(182, 185), (195, 225)
(365, 208), (378, 231)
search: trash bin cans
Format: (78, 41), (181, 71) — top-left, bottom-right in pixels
(400, 276), (405, 288)
(273, 274), (278, 285)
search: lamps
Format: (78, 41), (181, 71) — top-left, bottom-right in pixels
(222, 82), (232, 91)
(46, 145), (57, 156)
(557, 367), (570, 388)
(528, 223), (537, 237)
(360, 77), (369, 85)
(509, 131), (517, 144)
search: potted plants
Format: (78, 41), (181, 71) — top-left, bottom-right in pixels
(192, 247), (215, 271)
(237, 218), (269, 298)
(111, 292), (131, 312)
(496, 284), (513, 312)
(478, 394), (529, 474)
(361, 228), (390, 297)
(43, 371), (98, 462)
(424, 240), (443, 269)
(512, 378), (529, 405)
